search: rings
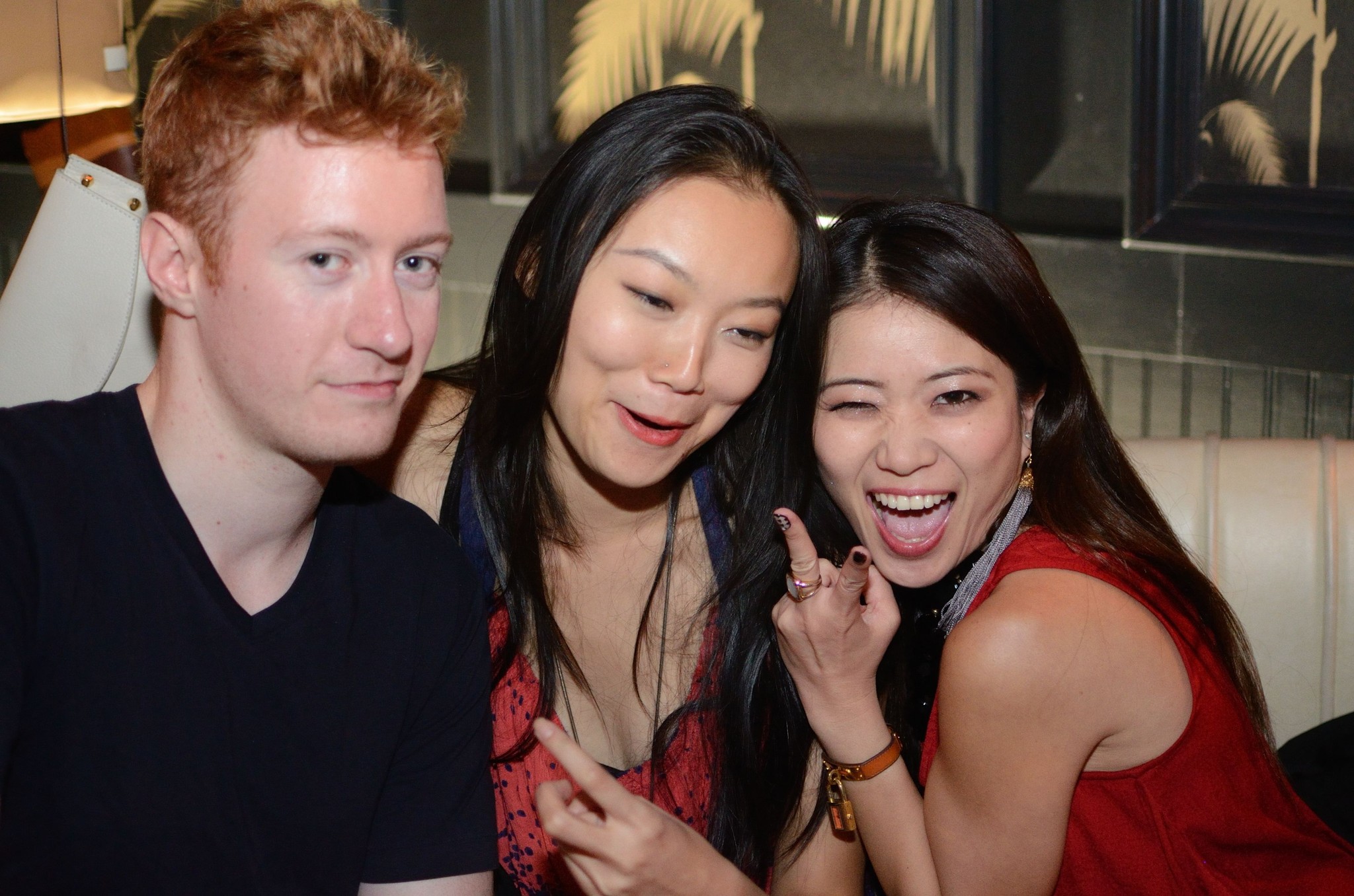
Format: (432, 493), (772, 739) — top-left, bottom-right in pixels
(786, 573), (822, 601)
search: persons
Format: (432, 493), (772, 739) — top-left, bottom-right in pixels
(376, 83), (866, 896)
(771, 200), (1354, 896)
(0, 0), (497, 896)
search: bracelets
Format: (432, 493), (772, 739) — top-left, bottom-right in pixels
(821, 726), (903, 832)
